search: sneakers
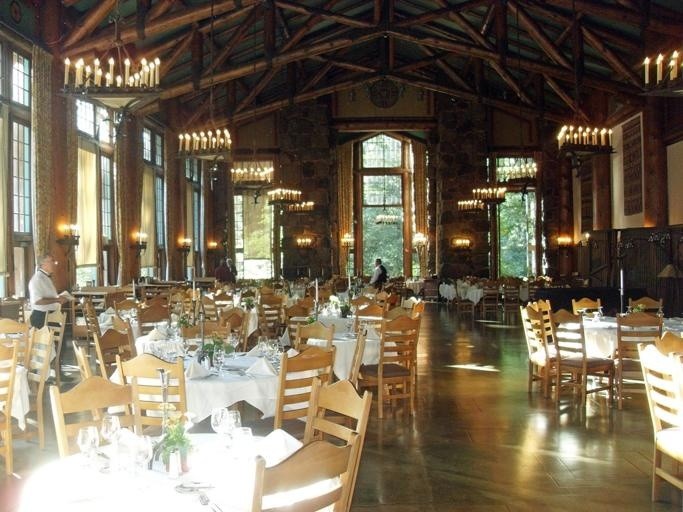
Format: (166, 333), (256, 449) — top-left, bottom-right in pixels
(52, 373), (75, 383)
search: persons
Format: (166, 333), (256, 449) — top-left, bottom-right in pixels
(368, 258), (386, 286)
(27, 251), (69, 383)
(225, 258), (237, 283)
(214, 257), (232, 283)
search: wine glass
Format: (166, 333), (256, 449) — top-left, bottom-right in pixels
(78, 406), (254, 512)
(140, 332), (280, 376)
(578, 306), (605, 326)
